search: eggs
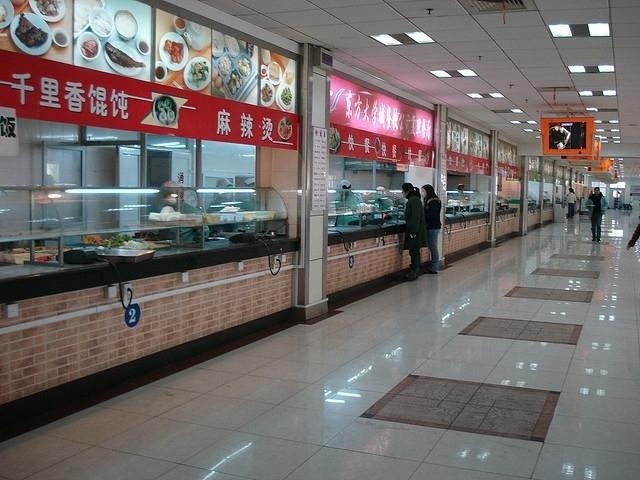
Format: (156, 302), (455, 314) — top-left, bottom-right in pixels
(211, 68), (222, 87)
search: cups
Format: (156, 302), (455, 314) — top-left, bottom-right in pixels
(357, 201), (377, 212)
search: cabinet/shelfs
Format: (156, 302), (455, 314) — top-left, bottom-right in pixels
(328, 189), (408, 231)
(446, 190), (486, 215)
(0, 187), (289, 271)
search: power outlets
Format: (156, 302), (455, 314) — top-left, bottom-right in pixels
(347, 242), (353, 249)
(121, 283), (133, 298)
(274, 255), (278, 264)
(380, 237), (384, 242)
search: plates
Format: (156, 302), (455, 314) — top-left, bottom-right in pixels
(276, 118), (293, 140)
(375, 138), (381, 155)
(0, 1), (298, 113)
(152, 95), (179, 128)
(328, 127), (341, 152)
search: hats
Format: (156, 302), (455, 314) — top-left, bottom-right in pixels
(341, 180), (351, 187)
(245, 177), (255, 185)
(217, 177), (233, 187)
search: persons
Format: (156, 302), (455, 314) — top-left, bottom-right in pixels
(629, 193), (633, 210)
(549, 123), (571, 149)
(626, 223), (640, 250)
(335, 180), (441, 280)
(145, 177), (255, 243)
(585, 187), (609, 244)
(566, 188), (577, 217)
(588, 191), (593, 200)
